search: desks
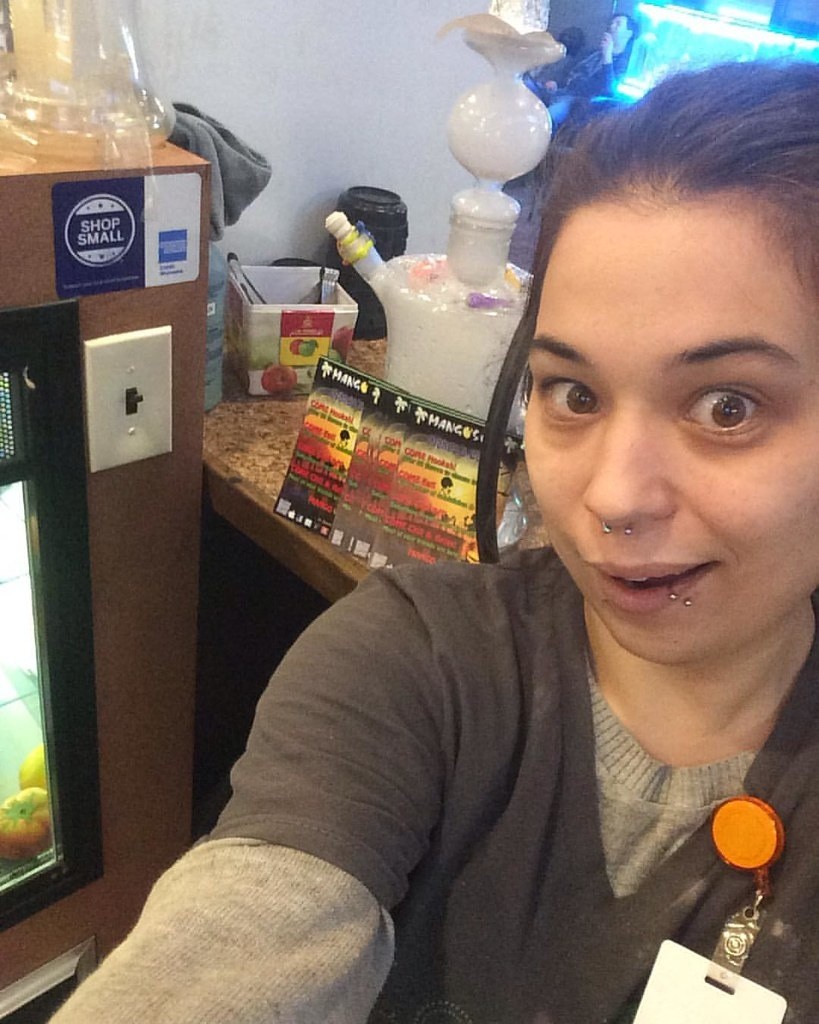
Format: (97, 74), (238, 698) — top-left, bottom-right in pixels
(200, 337), (386, 605)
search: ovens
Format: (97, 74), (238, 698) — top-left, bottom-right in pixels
(0, 139), (197, 1023)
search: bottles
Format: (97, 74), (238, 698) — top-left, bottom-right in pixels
(204, 241), (228, 412)
(1, 0), (180, 162)
(327, 185), (409, 340)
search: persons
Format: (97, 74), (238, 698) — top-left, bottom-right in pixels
(45, 59), (819, 1024)
(526, 15), (634, 134)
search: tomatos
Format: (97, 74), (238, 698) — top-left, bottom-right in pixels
(0, 745), (51, 863)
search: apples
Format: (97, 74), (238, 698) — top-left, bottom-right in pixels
(329, 327), (353, 364)
(260, 365), (297, 392)
(290, 339), (316, 356)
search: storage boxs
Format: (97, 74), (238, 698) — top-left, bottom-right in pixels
(222, 265), (359, 397)
